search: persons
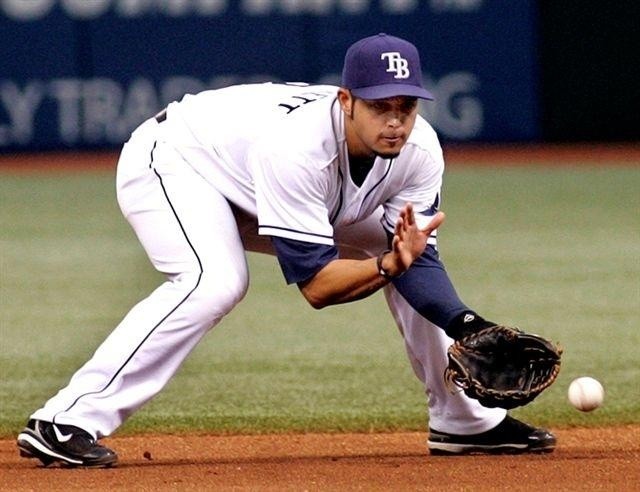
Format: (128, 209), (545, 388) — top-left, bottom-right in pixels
(16, 33), (561, 468)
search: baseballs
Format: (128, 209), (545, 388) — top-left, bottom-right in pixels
(568, 376), (604, 411)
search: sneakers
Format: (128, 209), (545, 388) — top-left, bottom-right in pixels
(17, 419), (117, 466)
(428, 415), (555, 455)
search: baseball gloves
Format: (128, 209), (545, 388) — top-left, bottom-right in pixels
(443, 324), (562, 410)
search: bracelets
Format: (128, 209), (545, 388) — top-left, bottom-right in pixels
(376, 249), (397, 281)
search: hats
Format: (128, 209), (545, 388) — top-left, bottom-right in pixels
(342, 32), (434, 99)
